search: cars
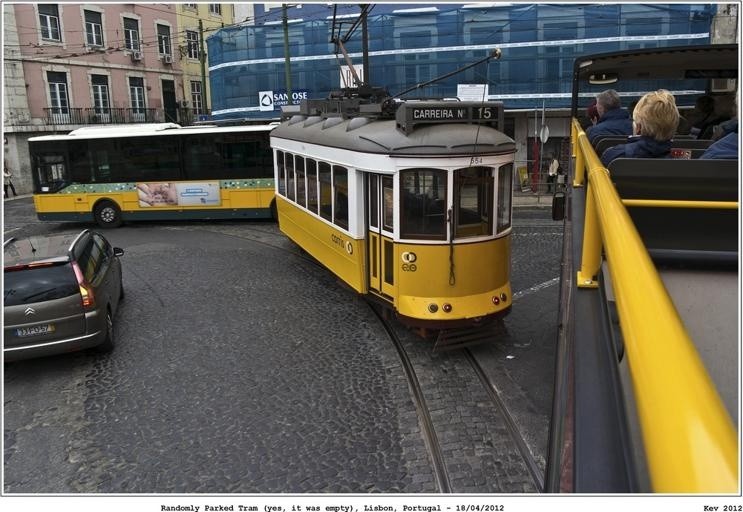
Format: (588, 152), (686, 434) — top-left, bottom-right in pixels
(4, 228), (124, 367)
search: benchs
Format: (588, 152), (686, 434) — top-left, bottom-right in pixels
(590, 130), (738, 258)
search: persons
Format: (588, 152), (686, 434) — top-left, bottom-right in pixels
(580, 88), (737, 168)
(4, 167), (18, 198)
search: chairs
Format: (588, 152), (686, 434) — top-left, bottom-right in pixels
(12, 278), (34, 292)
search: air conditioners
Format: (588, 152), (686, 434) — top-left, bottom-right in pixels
(131, 51), (141, 62)
(162, 56), (171, 64)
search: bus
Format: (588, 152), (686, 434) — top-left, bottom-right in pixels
(268, 49), (527, 327)
(544, 43), (738, 494)
(28, 123), (278, 228)
